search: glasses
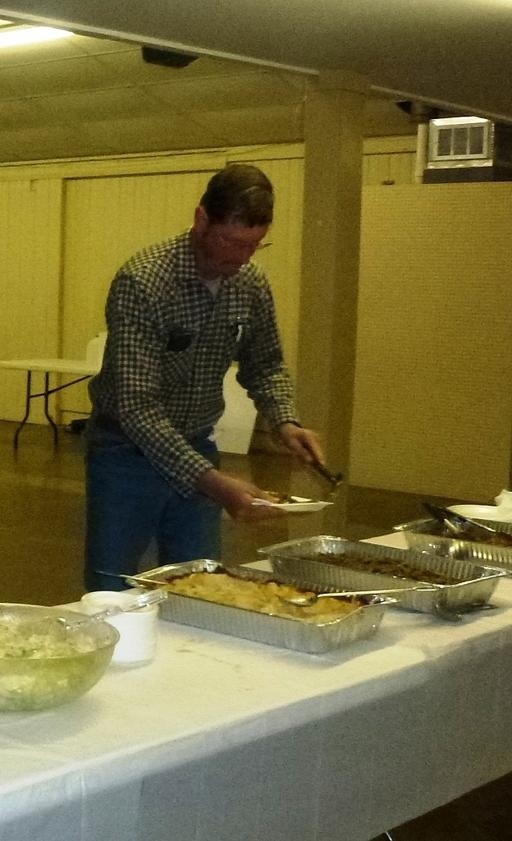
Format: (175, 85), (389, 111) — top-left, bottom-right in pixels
(208, 224), (273, 249)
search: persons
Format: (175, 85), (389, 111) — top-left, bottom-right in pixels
(84, 163), (327, 592)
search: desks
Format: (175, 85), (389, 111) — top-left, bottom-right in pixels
(0, 532), (511, 841)
(0, 359), (96, 447)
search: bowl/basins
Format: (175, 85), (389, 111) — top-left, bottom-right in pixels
(1, 602), (121, 712)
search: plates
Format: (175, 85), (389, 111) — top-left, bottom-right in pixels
(265, 490), (332, 515)
(442, 505), (512, 524)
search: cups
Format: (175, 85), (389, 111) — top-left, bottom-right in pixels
(81, 587), (160, 666)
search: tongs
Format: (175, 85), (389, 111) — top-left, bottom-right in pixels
(417, 500), (512, 544)
